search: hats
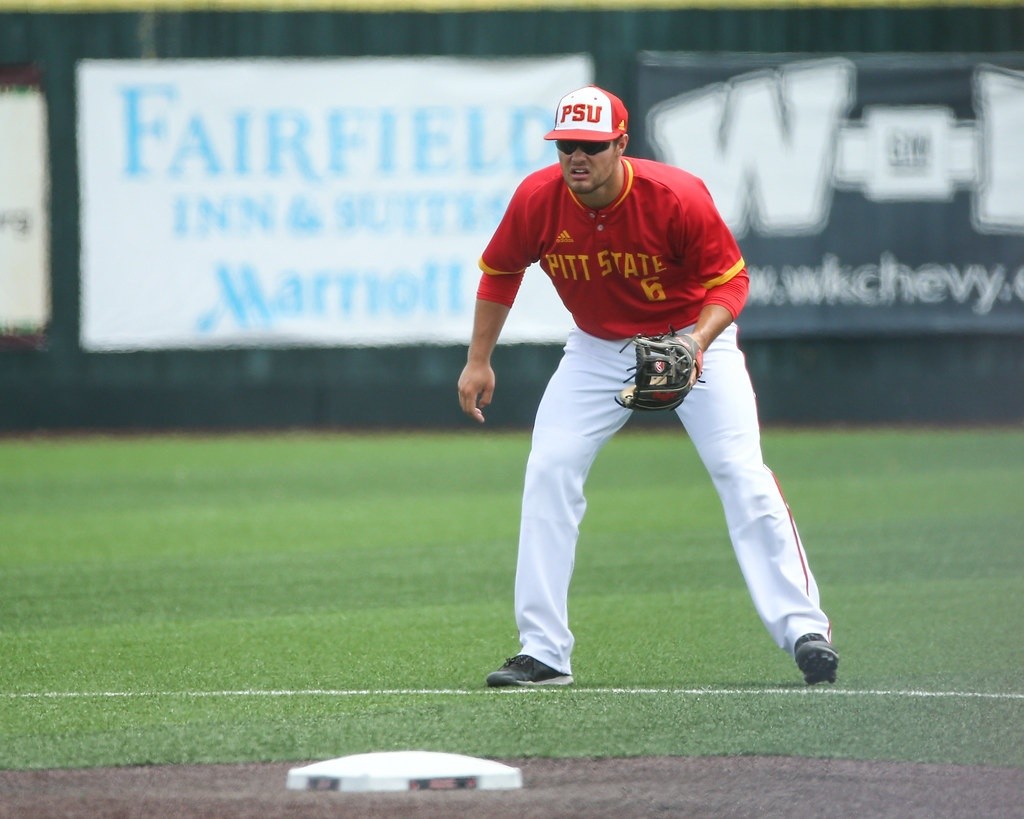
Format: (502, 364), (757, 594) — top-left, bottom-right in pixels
(544, 84), (629, 140)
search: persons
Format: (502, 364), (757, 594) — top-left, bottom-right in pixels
(457, 85), (842, 688)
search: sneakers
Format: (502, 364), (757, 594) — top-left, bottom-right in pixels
(487, 655), (574, 686)
(795, 633), (839, 685)
(555, 141), (610, 155)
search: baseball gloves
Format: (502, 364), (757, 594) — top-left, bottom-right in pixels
(619, 334), (704, 413)
